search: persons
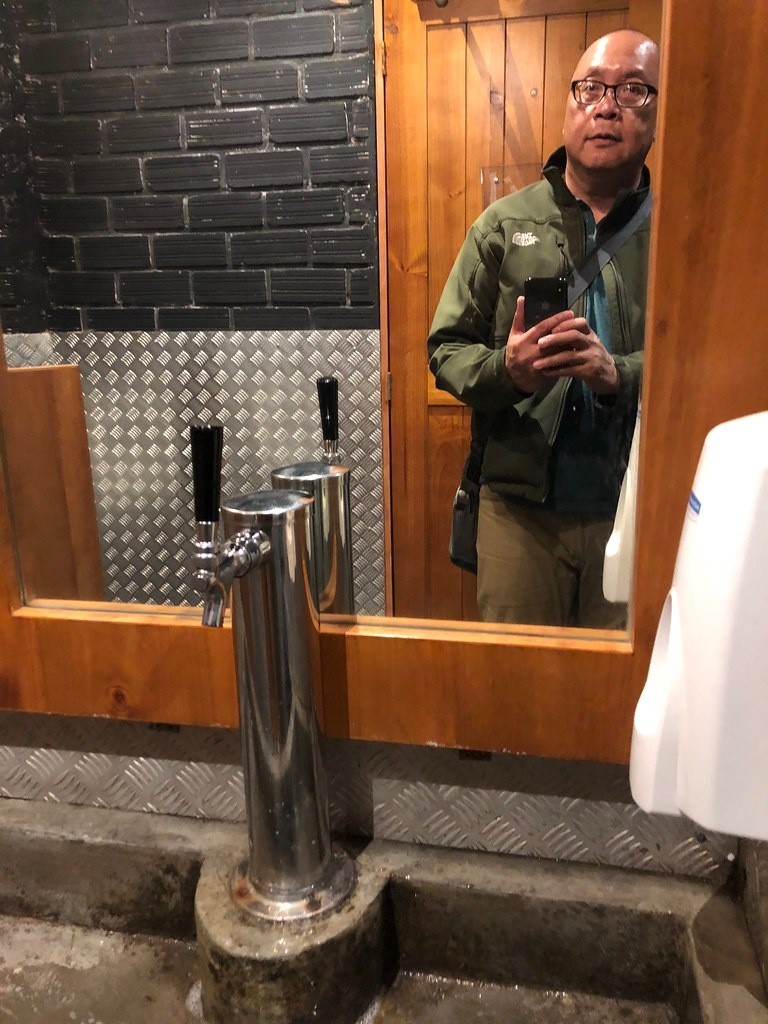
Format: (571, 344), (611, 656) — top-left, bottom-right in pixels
(428, 30), (659, 630)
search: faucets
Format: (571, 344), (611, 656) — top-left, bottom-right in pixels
(185, 421), (265, 631)
(318, 373), (344, 464)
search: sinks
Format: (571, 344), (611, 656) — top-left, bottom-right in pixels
(1, 823), (715, 1023)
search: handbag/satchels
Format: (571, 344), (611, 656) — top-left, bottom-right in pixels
(448, 486), (478, 574)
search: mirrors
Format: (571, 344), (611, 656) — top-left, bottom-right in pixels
(0, 0), (767, 766)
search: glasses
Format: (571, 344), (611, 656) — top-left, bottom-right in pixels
(572, 79), (657, 107)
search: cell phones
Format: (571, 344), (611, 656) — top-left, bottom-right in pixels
(523, 276), (569, 334)
